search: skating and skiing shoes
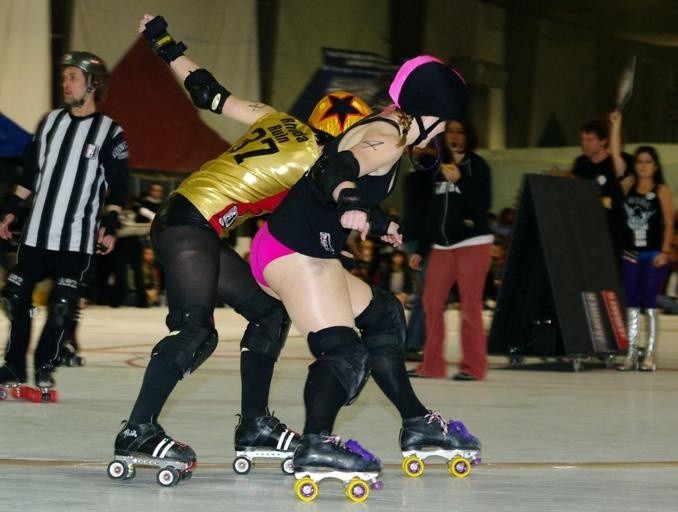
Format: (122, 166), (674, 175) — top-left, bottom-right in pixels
(293, 433), (383, 502)
(232, 407), (301, 473)
(29, 360), (58, 404)
(106, 416), (197, 487)
(399, 411), (482, 477)
(63, 342), (86, 367)
(0, 361), (29, 402)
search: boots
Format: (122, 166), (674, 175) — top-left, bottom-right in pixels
(618, 307), (639, 373)
(640, 308), (657, 372)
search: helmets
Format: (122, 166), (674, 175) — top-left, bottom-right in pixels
(308, 90), (374, 136)
(389, 55), (470, 120)
(54, 51), (109, 91)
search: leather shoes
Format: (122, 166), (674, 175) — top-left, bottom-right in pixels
(454, 371), (474, 381)
(407, 368), (420, 377)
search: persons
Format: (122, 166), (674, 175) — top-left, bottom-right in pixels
(128, 183), (164, 224)
(540, 123), (623, 267)
(138, 248), (161, 305)
(1, 53), (128, 402)
(497, 208), (516, 238)
(106, 14), (373, 486)
(251, 56), (483, 500)
(608, 112), (675, 371)
(382, 252), (408, 306)
(484, 242), (510, 301)
(409, 118), (493, 381)
